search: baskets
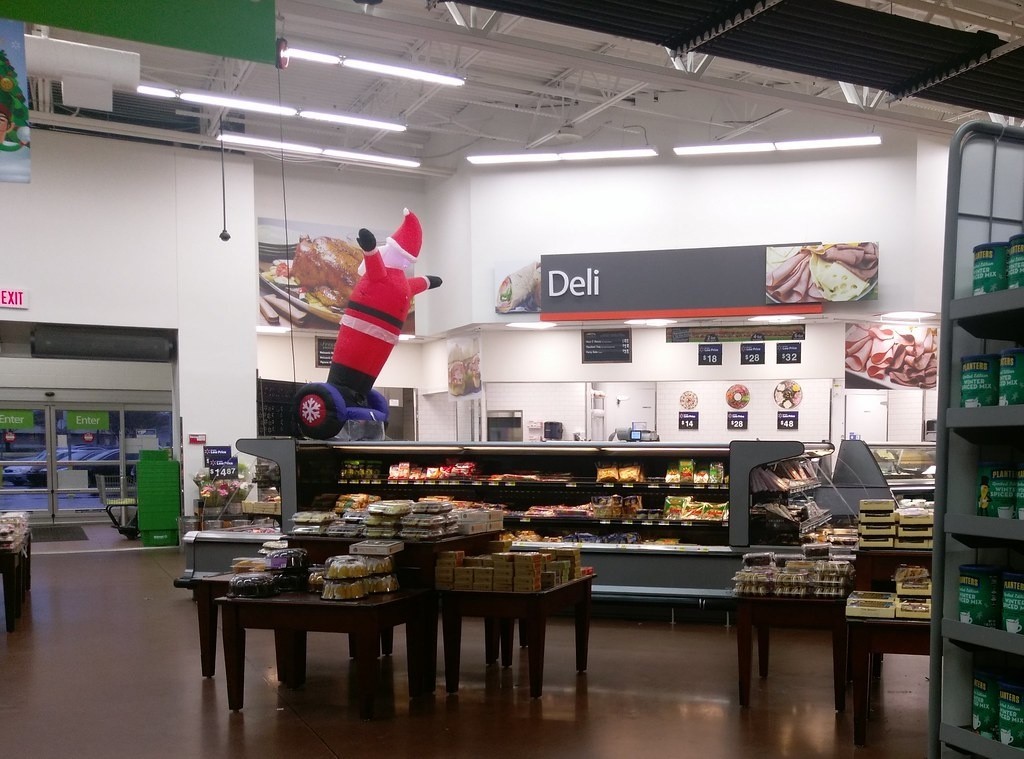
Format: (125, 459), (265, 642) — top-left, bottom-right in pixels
(136, 449), (180, 546)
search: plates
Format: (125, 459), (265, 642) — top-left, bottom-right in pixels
(256, 225), (303, 259)
(766, 243), (878, 303)
(845, 324), (939, 390)
(259, 259), (415, 323)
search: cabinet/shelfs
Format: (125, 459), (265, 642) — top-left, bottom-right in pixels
(929, 120), (1024, 759)
(235, 435), (836, 629)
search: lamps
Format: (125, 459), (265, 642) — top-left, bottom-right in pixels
(465, 134), (884, 167)
(138, 87), (421, 169)
(281, 48), (463, 88)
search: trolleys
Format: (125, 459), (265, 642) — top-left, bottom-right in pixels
(95, 472), (140, 539)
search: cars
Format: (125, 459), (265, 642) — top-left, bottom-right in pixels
(4, 443), (170, 486)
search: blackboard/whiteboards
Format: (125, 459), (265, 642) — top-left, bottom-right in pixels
(580, 327), (632, 363)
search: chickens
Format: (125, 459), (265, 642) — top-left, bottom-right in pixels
(276, 233), (364, 310)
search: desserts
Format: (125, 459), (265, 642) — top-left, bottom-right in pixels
(228, 493), (459, 599)
(728, 459), (930, 613)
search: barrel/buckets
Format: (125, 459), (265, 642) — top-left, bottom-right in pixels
(972, 242), (1008, 295)
(973, 664), (1000, 741)
(999, 348), (1024, 406)
(1016, 464), (1024, 520)
(1000, 571), (1024, 635)
(998, 680), (1024, 747)
(960, 354), (1000, 407)
(958, 564), (1003, 629)
(974, 461), (1017, 517)
(1009, 234), (1024, 288)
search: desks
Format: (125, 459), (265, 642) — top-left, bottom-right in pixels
(729, 592), (852, 718)
(175, 570), (280, 679)
(105, 499), (138, 539)
(436, 565), (597, 697)
(0, 528), (32, 633)
(214, 582), (425, 722)
(846, 542), (932, 743)
(279, 527), (507, 702)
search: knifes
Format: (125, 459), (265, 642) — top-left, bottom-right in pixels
(261, 278), (309, 326)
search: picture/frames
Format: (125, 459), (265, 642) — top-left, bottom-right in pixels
(580, 327), (632, 364)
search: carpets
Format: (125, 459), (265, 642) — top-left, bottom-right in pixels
(28, 526), (89, 542)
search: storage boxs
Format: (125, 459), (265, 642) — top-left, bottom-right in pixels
(846, 497), (935, 620)
(182, 530), (281, 601)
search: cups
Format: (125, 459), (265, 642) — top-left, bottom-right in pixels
(1006, 619), (1022, 633)
(997, 507), (1016, 519)
(973, 714), (981, 729)
(965, 399), (981, 407)
(1000, 396), (1008, 406)
(1001, 729), (1013, 745)
(960, 613), (973, 624)
